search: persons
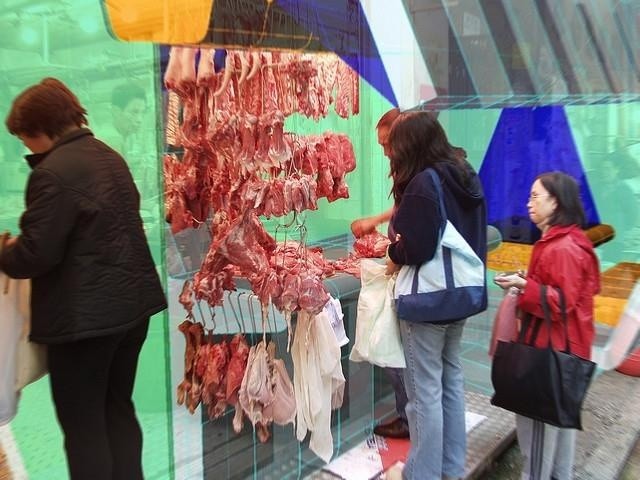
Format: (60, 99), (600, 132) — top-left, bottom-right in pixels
(494, 171), (601, 480)
(0, 78), (168, 480)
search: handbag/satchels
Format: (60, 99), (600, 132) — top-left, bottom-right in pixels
(490, 285), (598, 431)
(349, 259), (406, 368)
(0, 270), (48, 426)
(397, 219), (488, 324)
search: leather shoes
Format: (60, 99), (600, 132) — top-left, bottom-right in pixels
(374, 418), (409, 437)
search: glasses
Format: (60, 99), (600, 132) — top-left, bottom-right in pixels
(528, 194), (551, 201)
